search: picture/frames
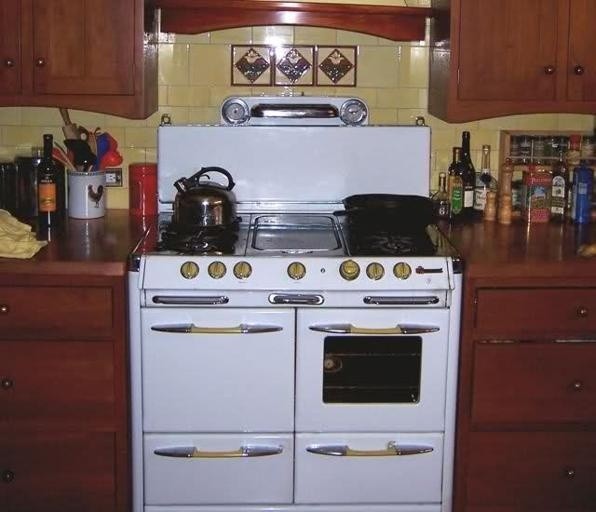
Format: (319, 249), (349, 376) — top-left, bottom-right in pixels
(230, 43), (359, 87)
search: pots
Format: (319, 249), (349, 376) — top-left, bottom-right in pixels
(334, 193), (437, 234)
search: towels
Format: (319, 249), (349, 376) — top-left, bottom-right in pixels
(0, 209), (48, 260)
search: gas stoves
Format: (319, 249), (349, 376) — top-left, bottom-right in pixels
(125, 210), (463, 255)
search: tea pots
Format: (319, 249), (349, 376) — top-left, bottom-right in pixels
(173, 166), (237, 228)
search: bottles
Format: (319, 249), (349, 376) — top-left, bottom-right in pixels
(128, 163), (158, 215)
(36, 134), (61, 229)
(508, 136), (594, 166)
(432, 131), (515, 226)
(0, 155), (34, 216)
(549, 160), (591, 225)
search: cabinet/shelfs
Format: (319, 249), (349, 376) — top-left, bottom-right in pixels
(428, 0), (596, 123)
(454, 277), (596, 512)
(498, 129), (596, 217)
(0, 0), (158, 120)
(0, 277), (131, 512)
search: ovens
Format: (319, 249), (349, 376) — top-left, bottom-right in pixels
(127, 254), (462, 512)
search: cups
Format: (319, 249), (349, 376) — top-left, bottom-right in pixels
(67, 172), (107, 220)
